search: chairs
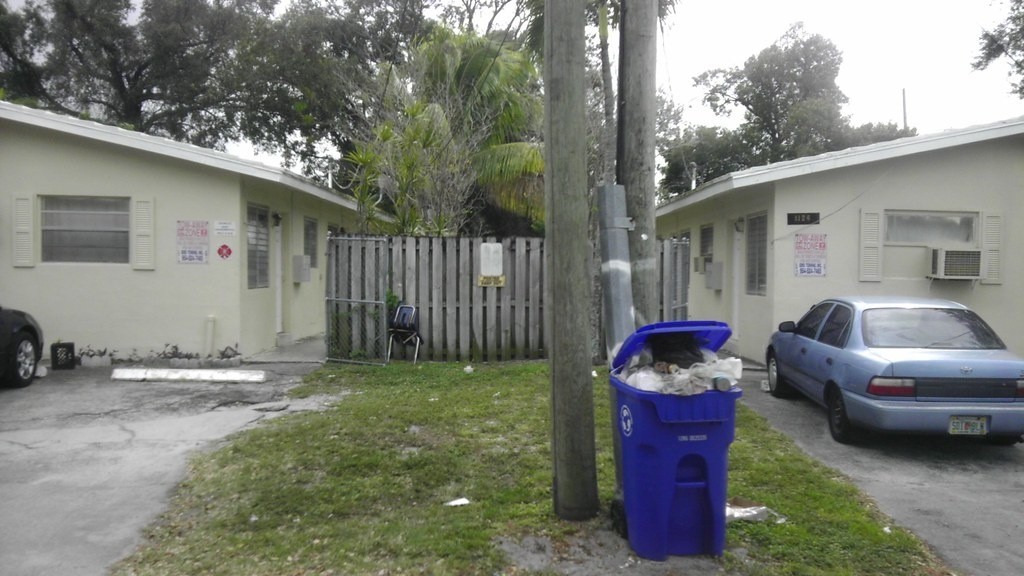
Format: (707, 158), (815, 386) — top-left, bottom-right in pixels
(372, 300), (424, 364)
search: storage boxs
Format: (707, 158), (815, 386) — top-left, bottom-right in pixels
(51, 342), (75, 371)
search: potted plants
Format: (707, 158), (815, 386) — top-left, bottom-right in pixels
(78, 343), (242, 368)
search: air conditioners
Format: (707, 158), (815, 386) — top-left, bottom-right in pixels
(926, 246), (984, 279)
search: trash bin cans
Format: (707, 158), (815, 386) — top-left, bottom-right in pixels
(606, 320), (745, 562)
(50, 342), (82, 371)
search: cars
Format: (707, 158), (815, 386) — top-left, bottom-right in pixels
(0, 306), (44, 388)
(765, 295), (1024, 446)
